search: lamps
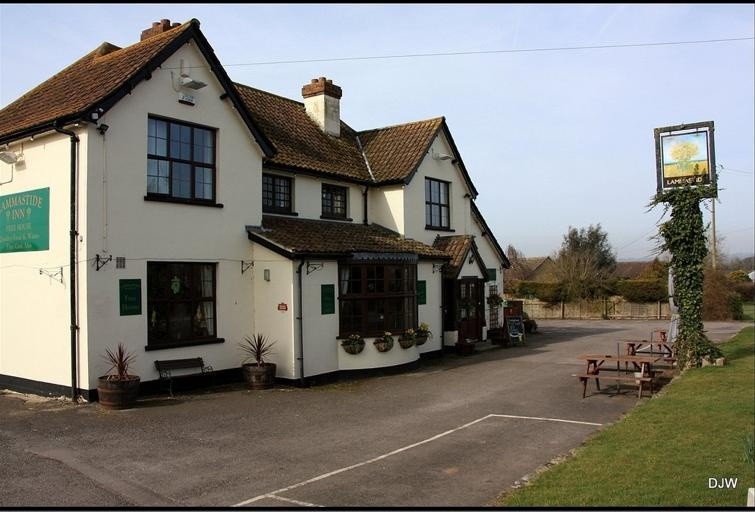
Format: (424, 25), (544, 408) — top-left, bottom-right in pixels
(180, 74), (207, 91)
(432, 151), (454, 162)
(0, 150), (24, 165)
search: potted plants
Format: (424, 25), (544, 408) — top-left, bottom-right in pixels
(95, 344), (142, 410)
(240, 334), (278, 390)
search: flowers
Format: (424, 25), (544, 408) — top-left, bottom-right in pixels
(342, 322), (429, 343)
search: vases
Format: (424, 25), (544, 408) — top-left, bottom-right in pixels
(344, 338), (428, 353)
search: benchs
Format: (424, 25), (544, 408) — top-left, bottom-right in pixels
(152, 358), (213, 400)
(571, 366), (664, 397)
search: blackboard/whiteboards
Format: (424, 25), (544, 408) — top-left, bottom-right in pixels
(507, 316), (524, 336)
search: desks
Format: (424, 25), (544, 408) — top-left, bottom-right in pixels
(576, 354), (662, 398)
(617, 338), (677, 354)
